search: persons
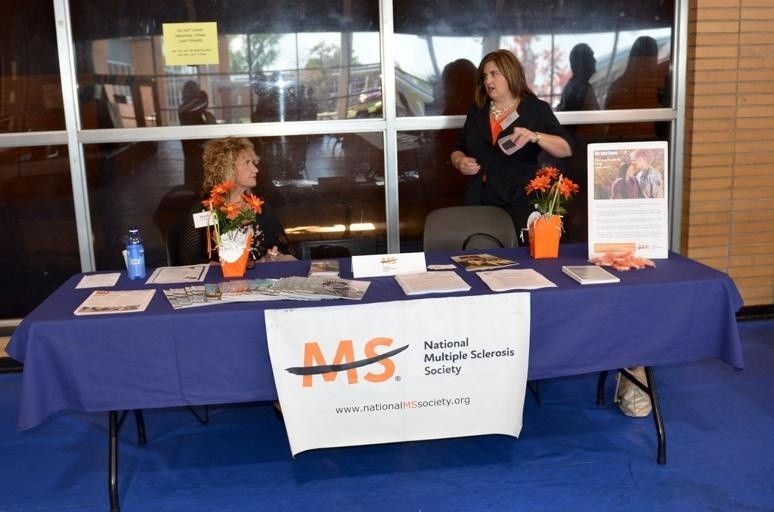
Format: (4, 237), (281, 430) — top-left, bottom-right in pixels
(610, 163), (639, 200)
(629, 150), (664, 198)
(247, 72), (319, 180)
(180, 135), (300, 265)
(177, 80), (216, 185)
(604, 36), (669, 141)
(450, 49), (578, 242)
(556, 42), (601, 142)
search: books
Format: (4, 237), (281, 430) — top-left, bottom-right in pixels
(561, 265), (620, 286)
(162, 275), (371, 310)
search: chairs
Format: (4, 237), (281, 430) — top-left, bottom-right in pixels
(163, 223), (186, 267)
(421, 204), (543, 406)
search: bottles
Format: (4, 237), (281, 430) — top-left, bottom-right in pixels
(126, 226), (145, 280)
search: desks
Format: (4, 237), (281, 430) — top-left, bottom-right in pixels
(5, 239), (749, 511)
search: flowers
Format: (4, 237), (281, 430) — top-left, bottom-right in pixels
(524, 168), (579, 214)
(203, 177), (263, 232)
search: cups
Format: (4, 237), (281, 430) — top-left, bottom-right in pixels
(121, 249), (127, 271)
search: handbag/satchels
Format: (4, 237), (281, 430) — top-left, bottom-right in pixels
(613, 365), (654, 419)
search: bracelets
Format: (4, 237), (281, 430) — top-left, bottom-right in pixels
(534, 130), (540, 145)
